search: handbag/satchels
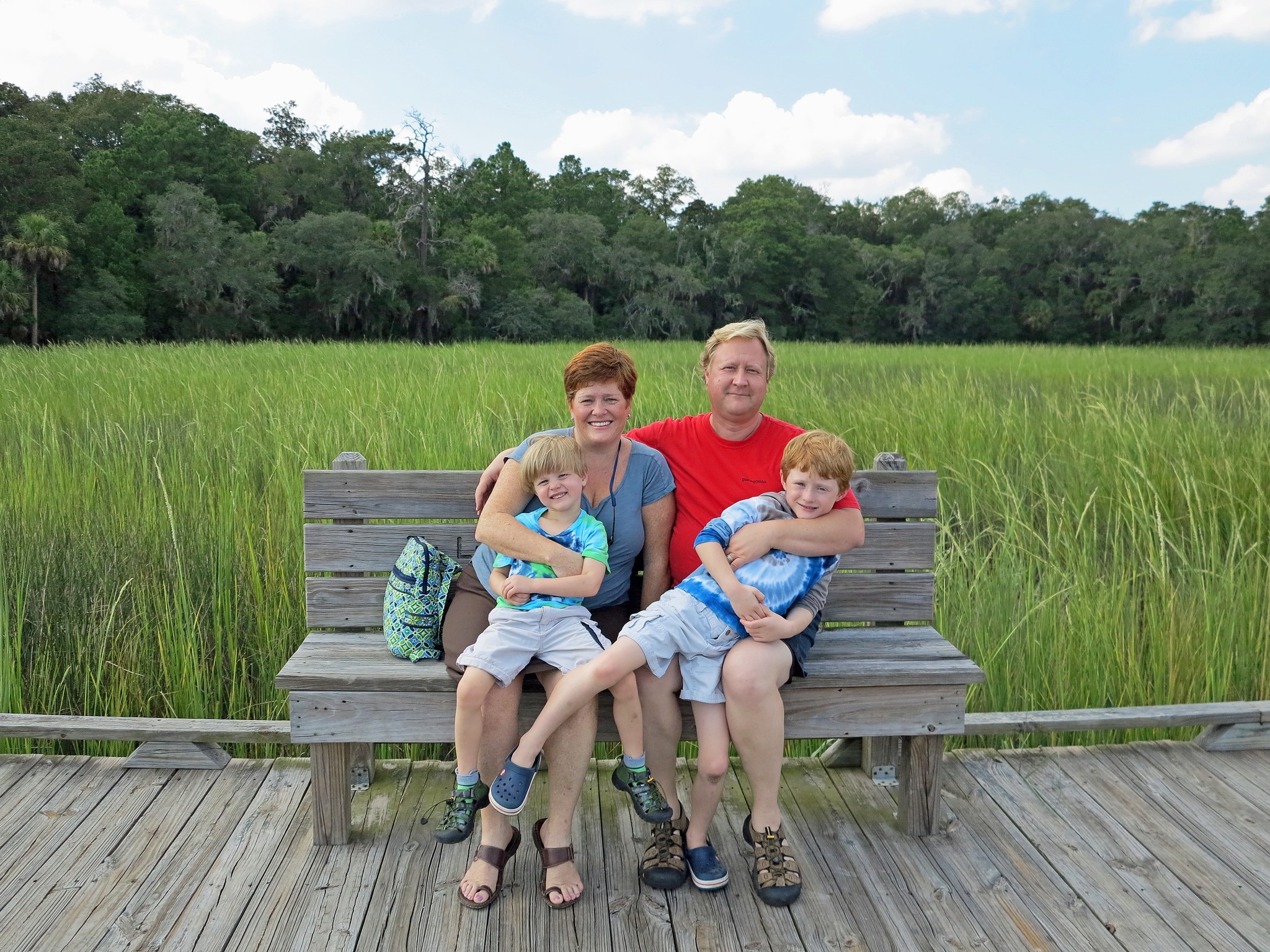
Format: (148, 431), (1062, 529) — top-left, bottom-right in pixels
(382, 535), (462, 663)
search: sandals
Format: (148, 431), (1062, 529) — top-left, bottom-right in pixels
(742, 814), (802, 907)
(489, 744), (540, 817)
(682, 828), (729, 890)
(639, 798), (689, 889)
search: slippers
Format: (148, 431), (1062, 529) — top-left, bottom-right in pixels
(457, 825), (521, 910)
(532, 818), (581, 909)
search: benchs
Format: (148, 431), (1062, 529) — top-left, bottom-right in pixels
(276, 452), (987, 846)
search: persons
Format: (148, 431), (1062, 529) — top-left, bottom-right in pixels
(440, 343), (676, 908)
(487, 431), (854, 891)
(421, 437), (675, 841)
(474, 316), (865, 912)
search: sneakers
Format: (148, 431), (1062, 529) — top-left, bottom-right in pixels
(611, 755), (673, 824)
(420, 771), (490, 844)
